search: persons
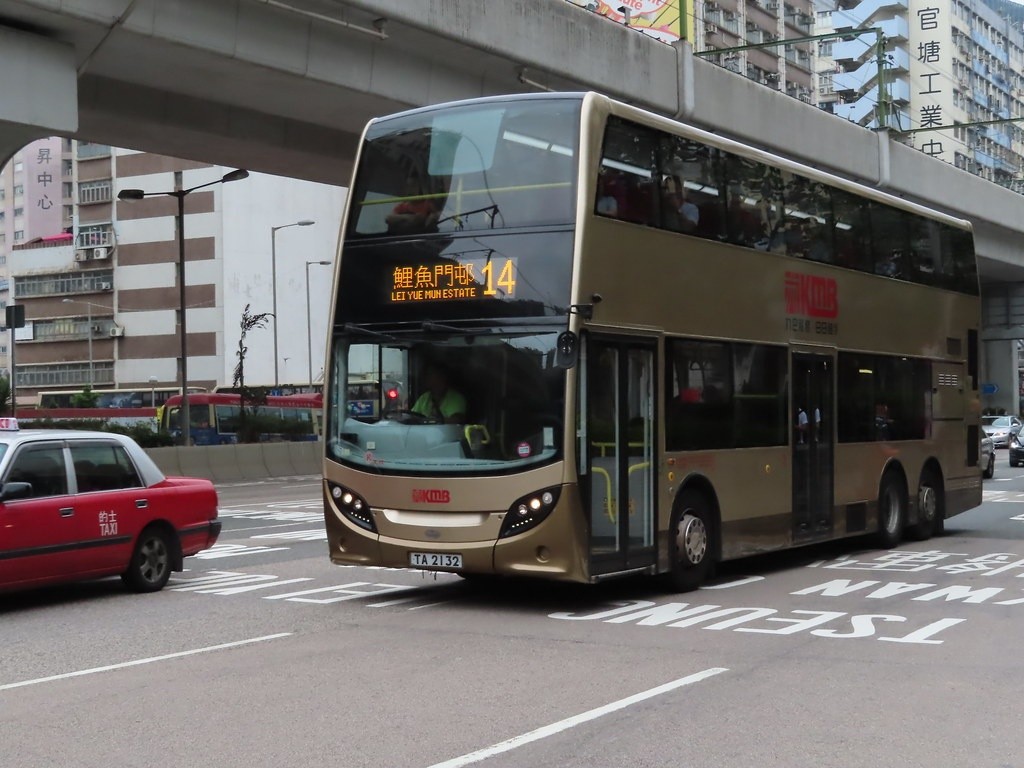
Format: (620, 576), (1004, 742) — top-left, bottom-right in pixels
(410, 368), (467, 424)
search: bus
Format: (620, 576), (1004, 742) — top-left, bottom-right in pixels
(35, 385), (211, 437)
(157, 391), (323, 443)
(210, 378), (387, 425)
(316, 91), (986, 594)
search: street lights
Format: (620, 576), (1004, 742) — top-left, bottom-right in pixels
(117, 168), (251, 445)
(63, 297), (96, 390)
(270, 217), (315, 393)
(304, 261), (335, 393)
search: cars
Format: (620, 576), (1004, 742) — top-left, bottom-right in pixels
(0, 416), (225, 595)
(1009, 424), (1024, 467)
(980, 428), (996, 476)
(982, 416), (1022, 447)
